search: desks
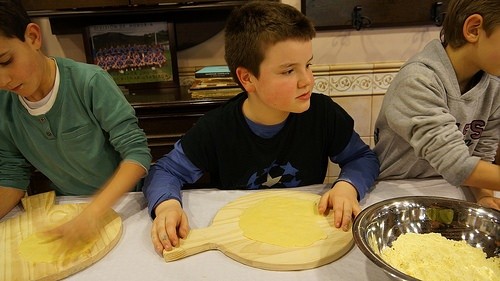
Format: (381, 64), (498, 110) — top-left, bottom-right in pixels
(0, 178), (500, 281)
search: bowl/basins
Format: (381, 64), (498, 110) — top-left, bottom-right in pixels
(353, 195), (500, 281)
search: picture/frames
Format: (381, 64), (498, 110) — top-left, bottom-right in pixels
(79, 14), (180, 90)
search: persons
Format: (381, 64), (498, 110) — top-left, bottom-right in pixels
(372, 0), (500, 212)
(0, 0), (152, 261)
(143, 0), (381, 257)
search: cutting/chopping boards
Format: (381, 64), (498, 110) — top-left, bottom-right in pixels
(0, 189), (123, 280)
(163, 184), (355, 271)
